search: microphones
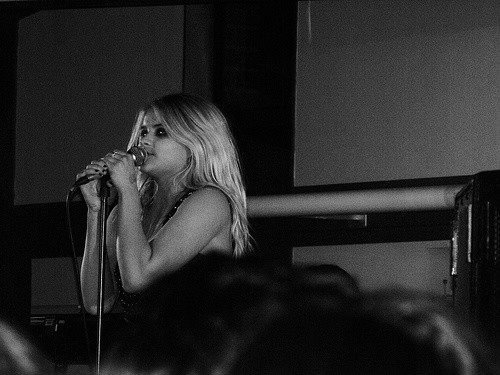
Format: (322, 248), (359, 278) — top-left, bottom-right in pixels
(71, 145), (148, 186)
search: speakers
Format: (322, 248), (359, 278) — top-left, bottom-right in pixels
(29, 255), (84, 314)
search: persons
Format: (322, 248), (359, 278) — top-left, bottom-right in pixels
(89, 248), (490, 375)
(75, 90), (258, 330)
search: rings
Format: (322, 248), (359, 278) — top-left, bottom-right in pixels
(111, 152), (118, 158)
